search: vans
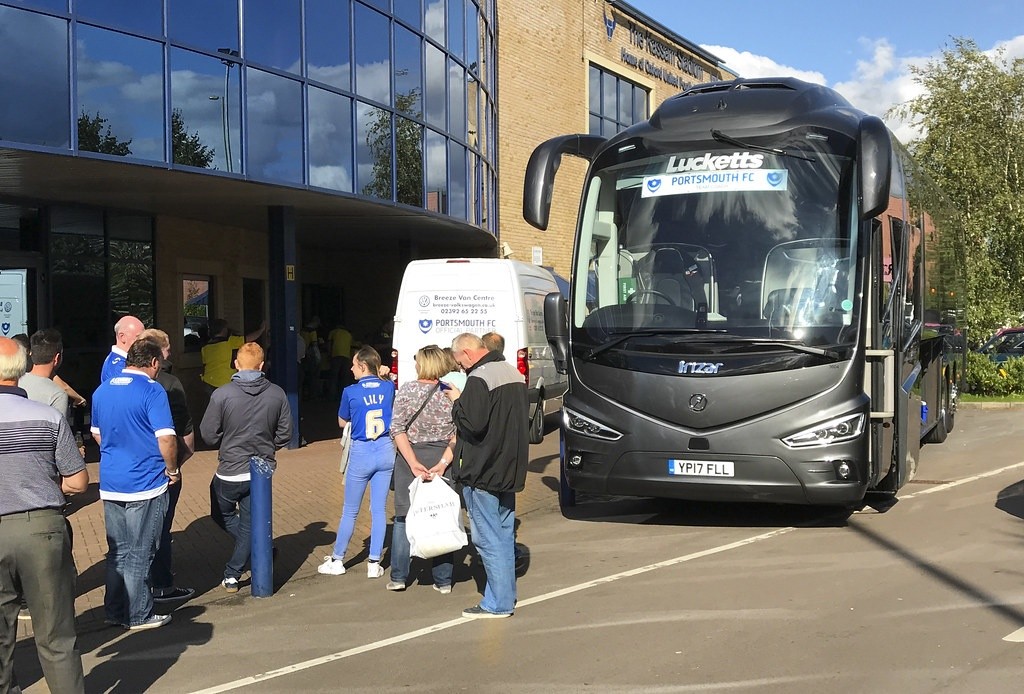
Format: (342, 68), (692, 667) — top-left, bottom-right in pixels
(390, 256), (566, 444)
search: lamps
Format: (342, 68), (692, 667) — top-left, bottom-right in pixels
(498, 242), (514, 257)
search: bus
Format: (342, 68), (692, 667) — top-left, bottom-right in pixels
(522, 75), (967, 511)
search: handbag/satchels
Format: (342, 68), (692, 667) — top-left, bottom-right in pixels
(406, 473), (469, 559)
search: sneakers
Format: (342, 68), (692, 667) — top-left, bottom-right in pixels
(154, 587), (196, 603)
(462, 605), (510, 618)
(433, 584), (452, 593)
(368, 562), (384, 578)
(123, 614), (172, 629)
(104, 618), (122, 624)
(273, 540), (279, 552)
(386, 581), (405, 590)
(222, 578), (238, 593)
(318, 556), (346, 575)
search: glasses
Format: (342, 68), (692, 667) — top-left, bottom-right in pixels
(414, 354), (422, 368)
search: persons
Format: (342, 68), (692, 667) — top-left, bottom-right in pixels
(202, 314), (393, 406)
(200, 343), (294, 592)
(0, 316), (195, 694)
(390, 333), (530, 619)
(317, 347), (397, 578)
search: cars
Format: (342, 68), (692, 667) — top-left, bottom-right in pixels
(976, 329), (1024, 364)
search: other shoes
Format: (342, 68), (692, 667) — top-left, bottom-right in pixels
(17, 608), (31, 619)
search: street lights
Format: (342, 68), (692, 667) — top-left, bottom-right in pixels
(200, 44), (244, 173)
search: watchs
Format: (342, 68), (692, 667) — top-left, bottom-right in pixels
(166, 468), (180, 476)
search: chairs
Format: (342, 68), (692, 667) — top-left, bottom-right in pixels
(634, 247), (694, 310)
(764, 285), (813, 322)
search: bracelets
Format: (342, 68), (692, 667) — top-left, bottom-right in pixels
(441, 459), (448, 466)
